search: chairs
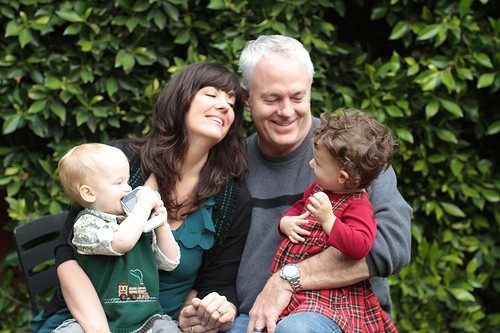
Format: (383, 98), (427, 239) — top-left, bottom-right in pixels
(14, 213), (69, 316)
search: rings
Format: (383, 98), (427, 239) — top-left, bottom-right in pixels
(190, 326), (193, 333)
(217, 309), (223, 316)
(254, 327), (263, 332)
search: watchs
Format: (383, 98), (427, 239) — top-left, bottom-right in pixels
(280, 264), (302, 293)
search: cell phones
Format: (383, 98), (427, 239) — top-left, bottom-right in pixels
(121, 187), (167, 232)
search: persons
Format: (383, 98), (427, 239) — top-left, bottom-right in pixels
(178, 35), (415, 333)
(270, 109), (399, 333)
(51, 142), (185, 333)
(29, 62), (253, 333)
